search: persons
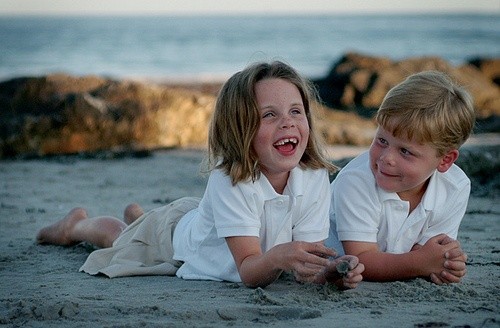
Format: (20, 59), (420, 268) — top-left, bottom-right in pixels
(319, 71), (476, 286)
(34, 60), (365, 291)
